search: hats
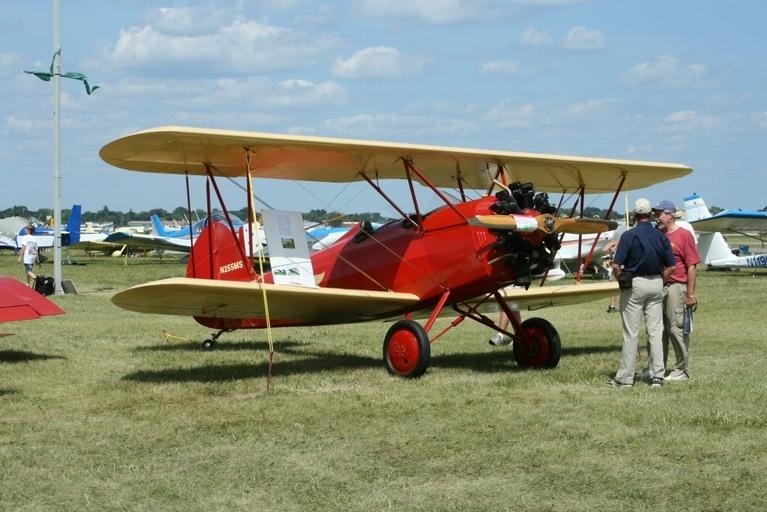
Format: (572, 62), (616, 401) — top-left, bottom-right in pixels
(634, 198), (651, 215)
(652, 200), (677, 214)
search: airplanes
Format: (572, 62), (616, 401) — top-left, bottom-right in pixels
(95, 123), (695, 379)
(0, 275), (66, 336)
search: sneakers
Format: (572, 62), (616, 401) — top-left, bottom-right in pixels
(607, 378), (635, 389)
(489, 333), (504, 348)
(649, 376), (664, 388)
(665, 368), (690, 381)
(606, 305), (618, 314)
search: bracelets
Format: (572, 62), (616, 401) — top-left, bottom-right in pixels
(686, 294), (695, 298)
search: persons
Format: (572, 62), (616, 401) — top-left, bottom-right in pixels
(15, 225), (41, 285)
(604, 198), (675, 389)
(488, 300), (521, 347)
(647, 200), (697, 382)
